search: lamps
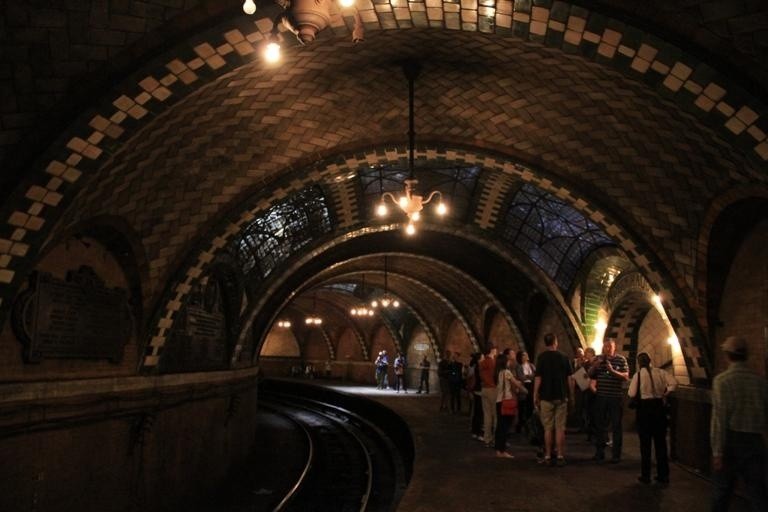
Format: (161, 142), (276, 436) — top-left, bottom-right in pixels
(240, 1), (365, 67)
(373, 67), (450, 236)
(274, 255), (402, 330)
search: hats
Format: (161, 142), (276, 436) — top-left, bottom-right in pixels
(722, 336), (746, 352)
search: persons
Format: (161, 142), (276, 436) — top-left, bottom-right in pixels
(375, 350), (432, 395)
(628, 351), (679, 486)
(709, 336), (767, 511)
(436, 332), (630, 465)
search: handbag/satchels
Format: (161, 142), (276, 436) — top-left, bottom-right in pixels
(501, 397), (519, 417)
(628, 394), (638, 408)
(522, 412), (544, 445)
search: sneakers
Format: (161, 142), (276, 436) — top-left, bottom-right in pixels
(537, 448), (565, 467)
(470, 431), (512, 458)
